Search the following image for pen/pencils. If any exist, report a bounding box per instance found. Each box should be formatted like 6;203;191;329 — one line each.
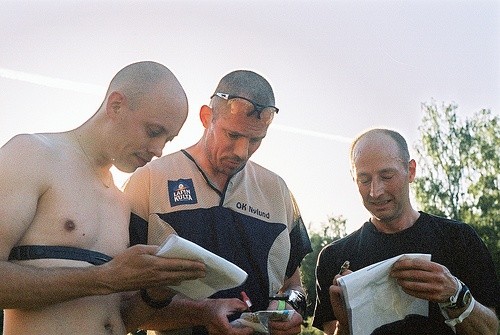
339;261;350;275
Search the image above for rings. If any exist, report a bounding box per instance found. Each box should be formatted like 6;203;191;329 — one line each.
277;299;285;310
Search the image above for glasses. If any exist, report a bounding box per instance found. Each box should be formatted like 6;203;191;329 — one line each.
211;93;280;126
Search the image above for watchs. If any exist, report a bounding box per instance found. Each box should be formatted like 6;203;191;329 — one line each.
438;276;473;309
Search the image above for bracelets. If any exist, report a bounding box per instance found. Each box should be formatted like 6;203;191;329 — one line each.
444;296;477;327
438;277;464;309
140;286;173;309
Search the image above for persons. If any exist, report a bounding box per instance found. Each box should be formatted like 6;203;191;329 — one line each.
311;128;500;335
119;70;313;335
1;61;207;335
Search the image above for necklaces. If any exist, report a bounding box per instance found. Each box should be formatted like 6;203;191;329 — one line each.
72;128;114;189
